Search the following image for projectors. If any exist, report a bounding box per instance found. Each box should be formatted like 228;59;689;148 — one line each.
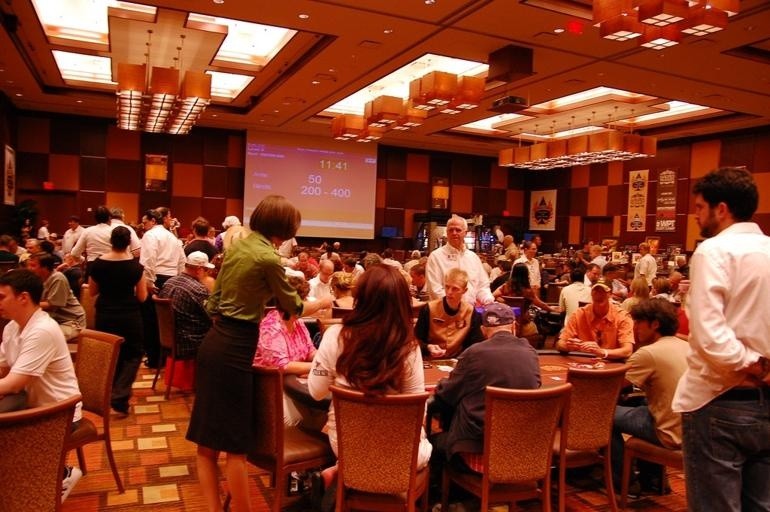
492;96;529;112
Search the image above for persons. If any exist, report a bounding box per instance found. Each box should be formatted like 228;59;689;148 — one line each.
14;218;64;270
140;211;187;369
422;218;495;306
492;263;561;337
1;269;82;503
89;226;147;415
544;260;575;288
71;207;115;284
362;247;432;292
533;235;543;255
184;217;218;262
324;271;357;319
302;259;335;349
156;207;171;229
556;282;635;394
510;241;540;298
158;251;215;361
111;208;141;258
63;216;87;253
278;236;369;279
308;264;431;509
414;267;487;361
559;270;593;326
600;298;692;498
0;235;19;269
494;224;519;280
27;251;86;340
186;195;335;512
253;276;329;493
431;303;542;511
671;168;769;511
577;240;629;296
620;245;690;312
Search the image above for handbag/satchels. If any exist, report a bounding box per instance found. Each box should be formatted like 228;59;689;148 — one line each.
528;304;542;321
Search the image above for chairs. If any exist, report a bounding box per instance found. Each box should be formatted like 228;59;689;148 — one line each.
413;302;427;318
328;384;429;512
65;283;100;363
66;328;126;494
547;283;567;302
502;296;539;348
332;307;353;318
441;383;572;512
620;432;684;509
553;364;633;512
303;318;324;350
0;394;83;512
222;363;336;512
0;261;17;272
151;294;197;399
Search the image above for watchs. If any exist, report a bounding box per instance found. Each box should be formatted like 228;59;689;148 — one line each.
602;349;608;358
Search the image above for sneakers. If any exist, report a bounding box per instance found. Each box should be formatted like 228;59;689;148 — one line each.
431;502;466;512
487;503;508;511
637;485;662;495
614;485;638;498
59;466;82;503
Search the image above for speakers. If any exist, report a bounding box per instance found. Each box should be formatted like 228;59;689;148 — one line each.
488;44;534;83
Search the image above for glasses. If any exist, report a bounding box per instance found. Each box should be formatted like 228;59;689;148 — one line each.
201;266;209;271
596;328;603;347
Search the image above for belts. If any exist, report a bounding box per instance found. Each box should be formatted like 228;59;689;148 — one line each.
713;384;769;403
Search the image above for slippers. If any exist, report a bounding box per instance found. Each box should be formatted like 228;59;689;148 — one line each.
309;470;324;510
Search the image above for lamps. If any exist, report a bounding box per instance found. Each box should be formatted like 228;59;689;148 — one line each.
364;96;403;129
491;93;671;170
105;6;229;135
592;0;739;51
438;76;485;116
408;71;457;111
392;101;428;131
354;127;385;143
329;114;364;143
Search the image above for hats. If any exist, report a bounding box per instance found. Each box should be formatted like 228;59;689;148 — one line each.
481;301;516;327
592;275;614;292
185;250;216;269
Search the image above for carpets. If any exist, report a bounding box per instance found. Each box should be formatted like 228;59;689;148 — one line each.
63;355;308;511
452;465;688;512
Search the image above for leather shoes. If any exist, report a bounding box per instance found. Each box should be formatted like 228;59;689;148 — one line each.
145;359;151;366
